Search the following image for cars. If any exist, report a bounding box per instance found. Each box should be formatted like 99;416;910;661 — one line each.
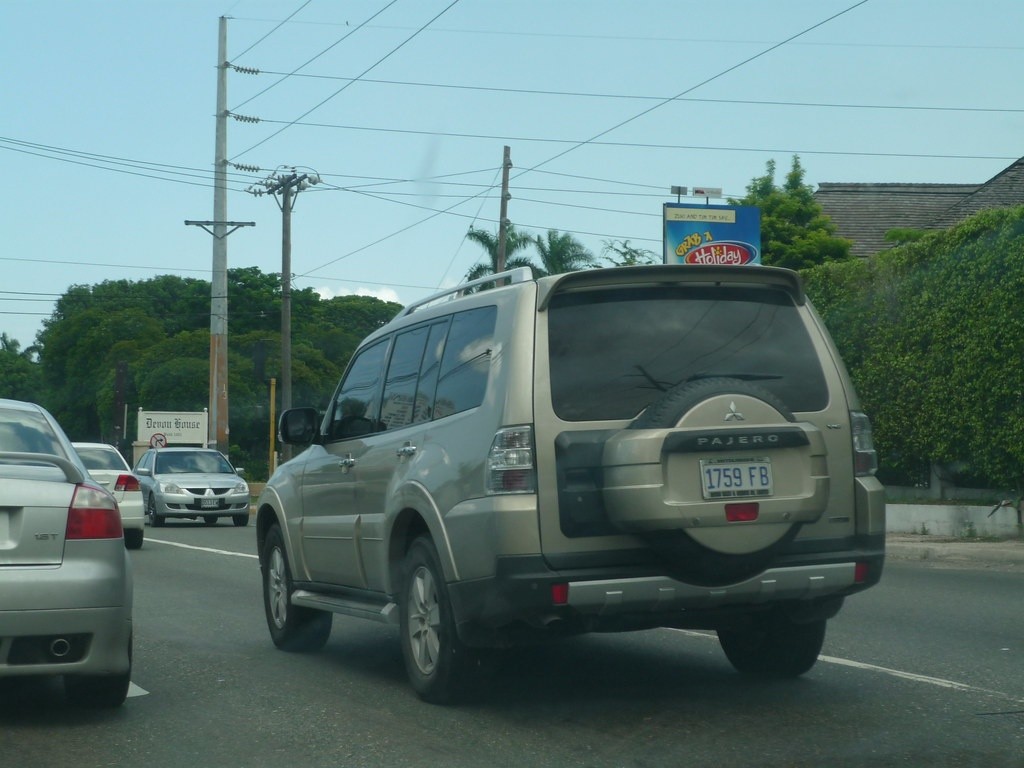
0;394;139;709
130;446;253;529
63;441;147;550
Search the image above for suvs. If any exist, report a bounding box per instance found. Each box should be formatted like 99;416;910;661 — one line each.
253;261;889;707
